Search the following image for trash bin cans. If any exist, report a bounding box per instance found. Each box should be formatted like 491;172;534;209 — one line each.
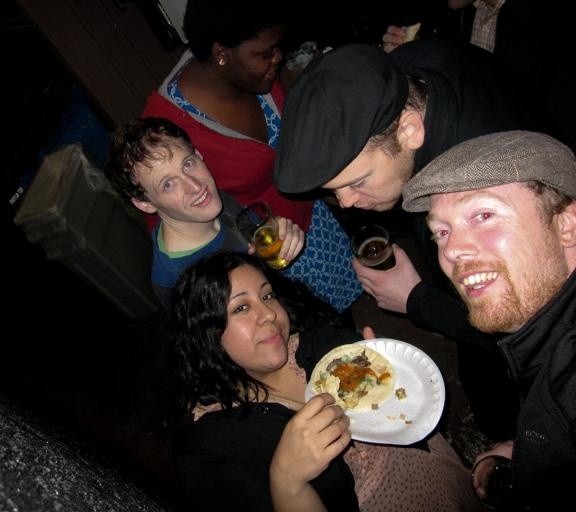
13;144;159;317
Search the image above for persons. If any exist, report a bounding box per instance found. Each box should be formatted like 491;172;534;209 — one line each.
107;1;576;512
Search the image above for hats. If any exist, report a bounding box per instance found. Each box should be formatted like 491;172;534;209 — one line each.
400;131;575;214
272;44;409;194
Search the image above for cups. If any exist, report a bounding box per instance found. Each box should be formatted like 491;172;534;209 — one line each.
350;224;395;270
234;201;291;272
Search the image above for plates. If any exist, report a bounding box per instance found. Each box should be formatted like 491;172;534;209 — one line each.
303;338;446;447
